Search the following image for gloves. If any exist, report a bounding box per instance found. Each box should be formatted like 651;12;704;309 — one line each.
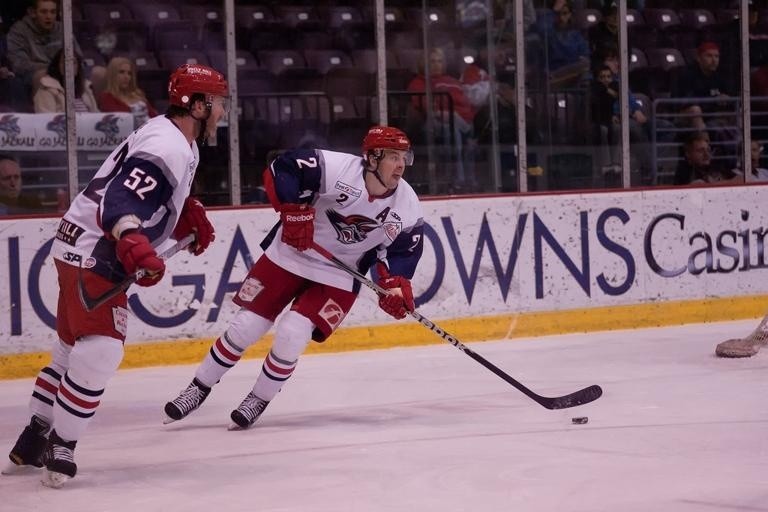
173;194;217;259
278;205;318;253
115;231;168;289
377;274;417;321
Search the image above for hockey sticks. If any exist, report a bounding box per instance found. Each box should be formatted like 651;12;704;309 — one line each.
77;233;195;310
311;242;602;410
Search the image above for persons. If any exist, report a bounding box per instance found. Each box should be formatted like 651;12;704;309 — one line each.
5;64;233;480
0;18;18;80
405;0;768;186
161;124;429;427
31;47;102;116
5;0;89;77
96;56;159;119
0;154;48;215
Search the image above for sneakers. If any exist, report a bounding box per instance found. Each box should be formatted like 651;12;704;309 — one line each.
163;376;221;421
42;427;81;477
8;414;52;469
228;389;272;430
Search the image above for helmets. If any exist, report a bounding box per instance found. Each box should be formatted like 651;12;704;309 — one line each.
165;62;230;112
358;123;413;158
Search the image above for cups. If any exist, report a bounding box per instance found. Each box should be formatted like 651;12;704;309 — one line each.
54;187;70;212
